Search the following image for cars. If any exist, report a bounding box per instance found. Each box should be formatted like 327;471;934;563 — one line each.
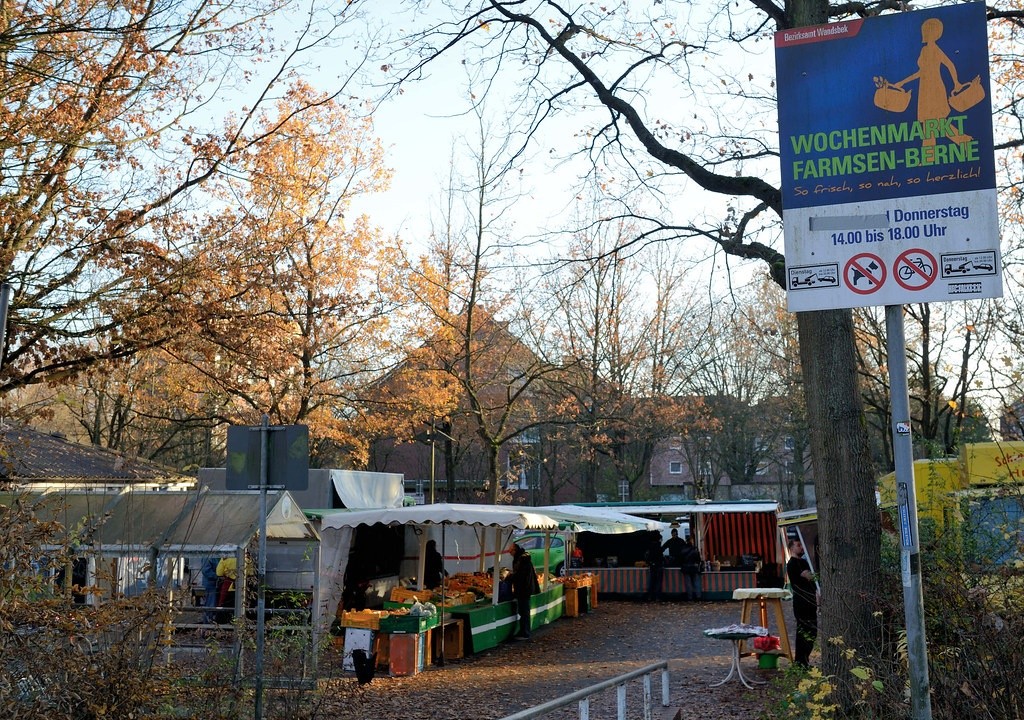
512;532;566;578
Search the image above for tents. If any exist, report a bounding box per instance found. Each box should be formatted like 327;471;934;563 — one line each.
537;503;672;575
312;501;559;663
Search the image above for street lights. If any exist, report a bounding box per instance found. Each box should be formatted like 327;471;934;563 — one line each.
413;416;455;503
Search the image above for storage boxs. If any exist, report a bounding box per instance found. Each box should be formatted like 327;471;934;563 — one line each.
340;608;388;631
565;574;599;589
379;614;429;634
427;612;441;628
389;586;433;604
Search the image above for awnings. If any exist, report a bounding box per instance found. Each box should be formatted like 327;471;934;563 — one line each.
778;505;820;591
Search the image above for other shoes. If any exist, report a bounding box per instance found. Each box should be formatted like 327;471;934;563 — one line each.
513;632;529;640
793;664;815;673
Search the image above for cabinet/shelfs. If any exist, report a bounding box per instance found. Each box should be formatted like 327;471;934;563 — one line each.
570;567;756;593
383;582;564;655
389;618;464;676
566;584;599;617
702;623;771;690
342;627;373;671
733;585;794;668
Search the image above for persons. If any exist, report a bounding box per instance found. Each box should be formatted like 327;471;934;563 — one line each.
649;535;664;603
787;540;817;667
199;557;220;638
424;540;448;589
341;575;372;611
215;551;256;629
678;535;703;603
507;543;542;641
662;528;687;565
